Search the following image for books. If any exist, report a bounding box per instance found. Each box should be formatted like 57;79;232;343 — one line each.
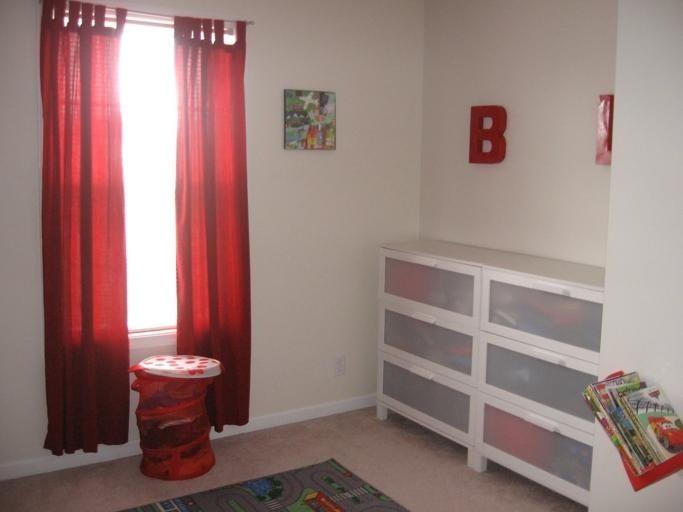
581;370;683;477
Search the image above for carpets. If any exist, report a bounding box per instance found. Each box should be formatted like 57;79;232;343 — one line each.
117;458;411;512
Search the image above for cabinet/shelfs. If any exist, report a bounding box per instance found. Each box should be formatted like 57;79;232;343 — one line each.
375;241;604;506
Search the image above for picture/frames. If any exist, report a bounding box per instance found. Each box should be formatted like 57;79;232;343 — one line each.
283;90;336;151
